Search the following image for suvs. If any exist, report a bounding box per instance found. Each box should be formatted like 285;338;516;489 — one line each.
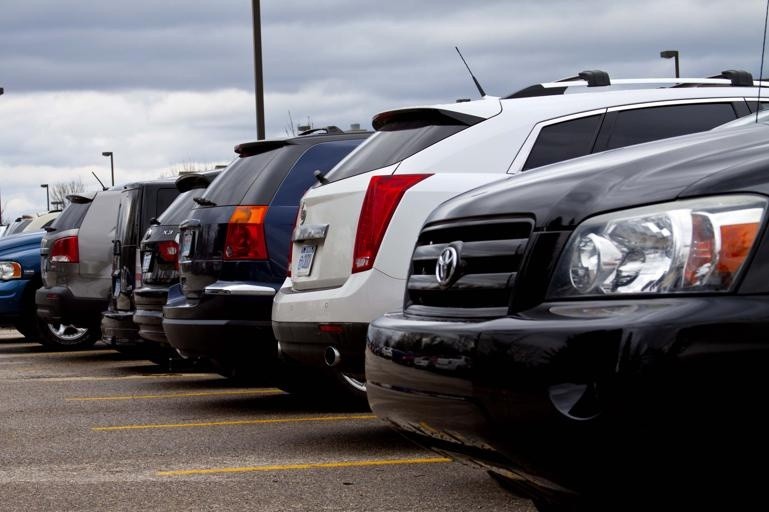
364;108;767;507
268;68;769;507
162;129;371;398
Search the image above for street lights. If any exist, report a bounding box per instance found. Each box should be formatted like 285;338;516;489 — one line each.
101;151;115;187
40;182;50;211
